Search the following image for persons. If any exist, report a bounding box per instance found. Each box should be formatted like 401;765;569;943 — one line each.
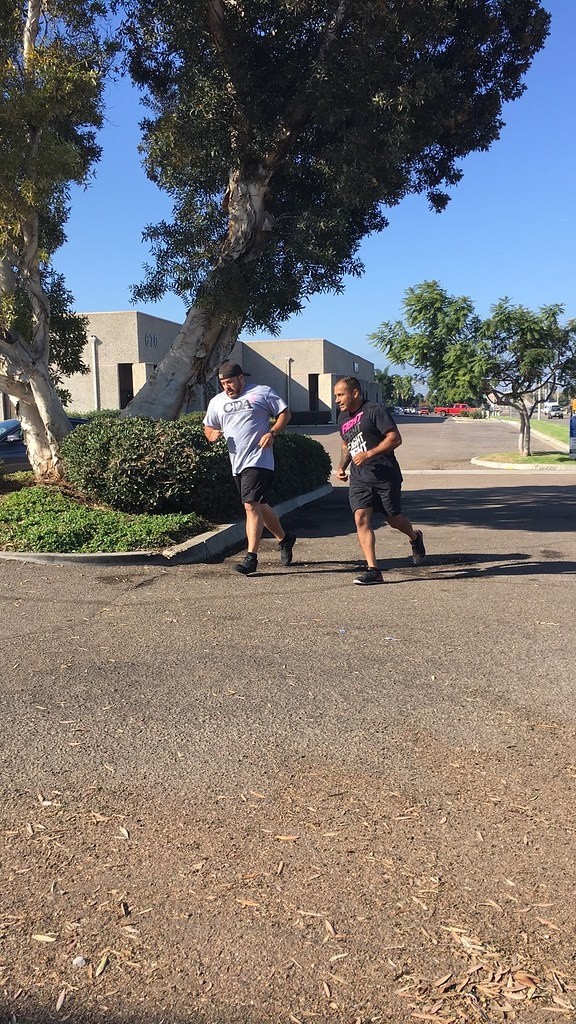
201;361;297;574
332;375;426;586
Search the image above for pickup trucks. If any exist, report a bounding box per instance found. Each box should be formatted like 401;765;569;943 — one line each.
435;403;479;417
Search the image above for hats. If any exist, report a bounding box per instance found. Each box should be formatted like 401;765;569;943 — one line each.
219;362;252;380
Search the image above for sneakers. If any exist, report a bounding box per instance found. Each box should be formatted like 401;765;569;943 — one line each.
410;530;425;565
232;555;258;574
278;534;296;566
353;568;384;584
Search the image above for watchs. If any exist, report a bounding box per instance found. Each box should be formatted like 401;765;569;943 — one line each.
268;429;279;440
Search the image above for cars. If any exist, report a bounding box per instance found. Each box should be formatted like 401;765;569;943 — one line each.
420;406;429;415
404;407;416;414
394;407;404;414
0;418;93;474
529;405;538;413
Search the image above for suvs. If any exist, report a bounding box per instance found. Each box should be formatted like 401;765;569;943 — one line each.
548;405;563;419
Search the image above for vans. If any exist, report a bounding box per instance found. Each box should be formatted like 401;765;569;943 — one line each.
542;403;560;415
